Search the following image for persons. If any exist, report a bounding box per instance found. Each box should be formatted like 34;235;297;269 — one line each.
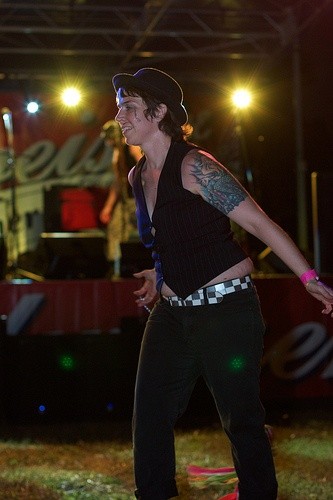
113;67;333;500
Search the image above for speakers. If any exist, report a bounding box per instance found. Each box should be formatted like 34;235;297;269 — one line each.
119;242;155;279
47;234;108;280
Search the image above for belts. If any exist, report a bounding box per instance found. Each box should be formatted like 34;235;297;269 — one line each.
162;275;253;306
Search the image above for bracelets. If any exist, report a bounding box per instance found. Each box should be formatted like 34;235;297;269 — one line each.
300;269;319;286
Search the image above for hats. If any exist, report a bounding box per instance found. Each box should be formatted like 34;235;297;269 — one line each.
112;68;189;127
